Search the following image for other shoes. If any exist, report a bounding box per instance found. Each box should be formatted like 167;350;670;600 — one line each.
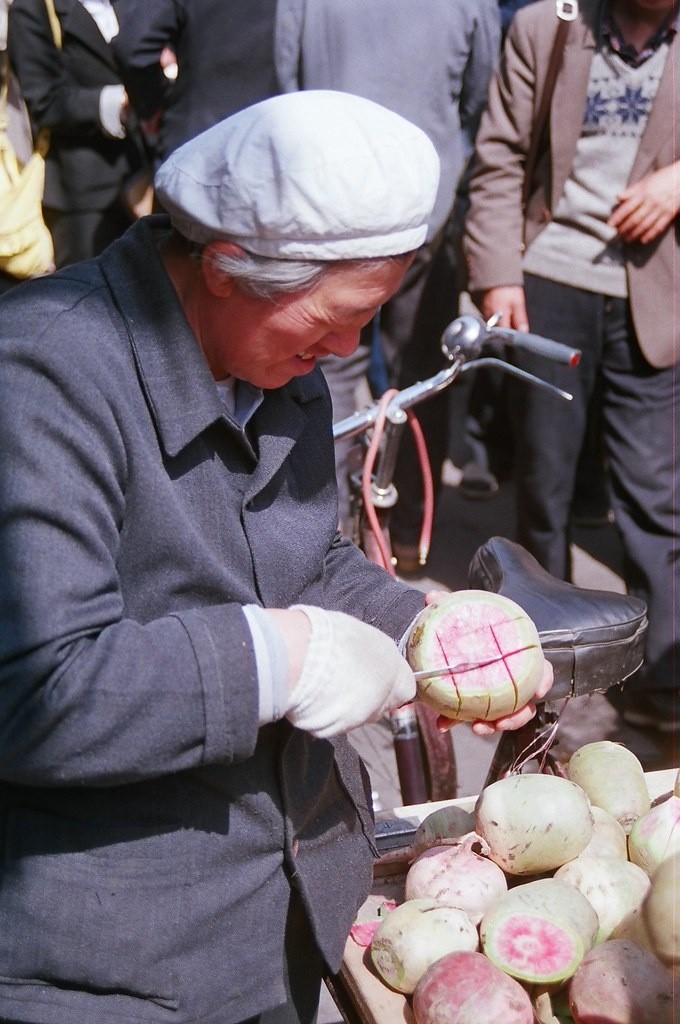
391;540;424;573
622;690;680;732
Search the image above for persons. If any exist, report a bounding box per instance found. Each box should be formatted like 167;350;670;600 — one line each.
0;91;554;1024
0;0;680;735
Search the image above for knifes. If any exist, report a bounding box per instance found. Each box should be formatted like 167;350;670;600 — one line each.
416;656;510;677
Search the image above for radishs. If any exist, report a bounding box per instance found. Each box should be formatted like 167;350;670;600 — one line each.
368;740;680;1024
407;590;544;722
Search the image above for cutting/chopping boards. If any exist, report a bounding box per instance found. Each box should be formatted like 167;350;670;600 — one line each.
342;767;680;1024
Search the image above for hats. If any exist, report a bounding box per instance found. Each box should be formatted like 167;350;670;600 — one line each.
154;90;441;260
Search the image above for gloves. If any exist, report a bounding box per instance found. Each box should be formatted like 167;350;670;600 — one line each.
282;604;417;739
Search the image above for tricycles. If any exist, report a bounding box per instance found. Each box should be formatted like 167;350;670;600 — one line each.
323;309;679;1024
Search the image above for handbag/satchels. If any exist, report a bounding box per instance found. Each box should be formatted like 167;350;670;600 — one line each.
1;115;56;279
